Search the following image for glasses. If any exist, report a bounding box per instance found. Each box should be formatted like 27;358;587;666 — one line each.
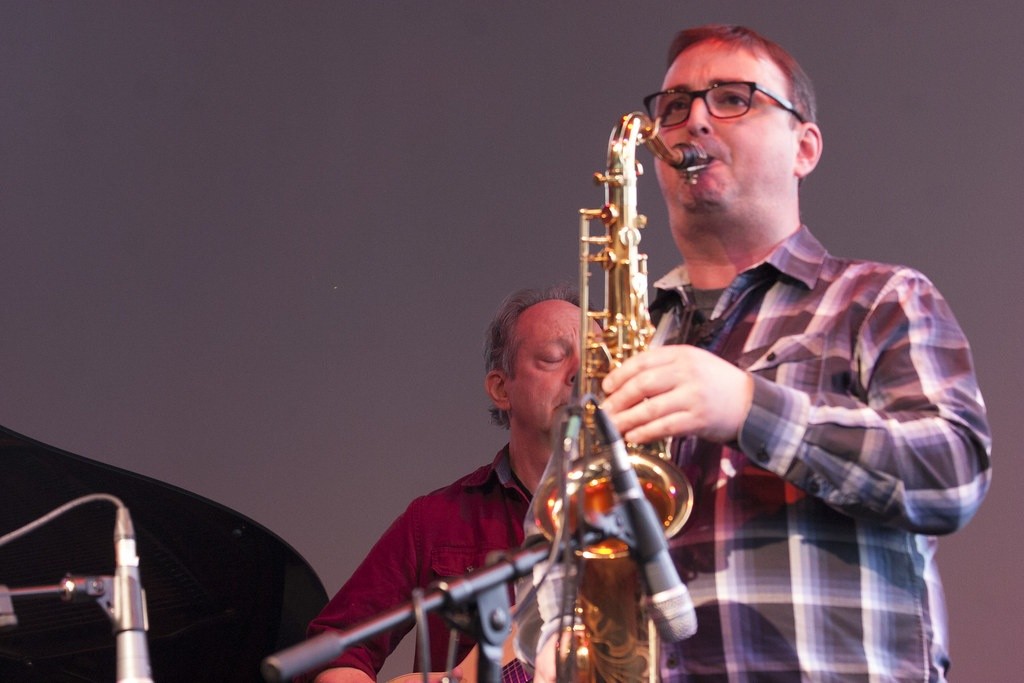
642;82;807;129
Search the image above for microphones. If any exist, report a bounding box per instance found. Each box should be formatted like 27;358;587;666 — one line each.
594;409;697;644
113;507;155;683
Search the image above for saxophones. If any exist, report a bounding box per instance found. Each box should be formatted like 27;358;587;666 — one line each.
551;110;710;681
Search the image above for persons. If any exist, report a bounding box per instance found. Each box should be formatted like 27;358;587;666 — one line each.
295;284;602;682
601;24;993;683
